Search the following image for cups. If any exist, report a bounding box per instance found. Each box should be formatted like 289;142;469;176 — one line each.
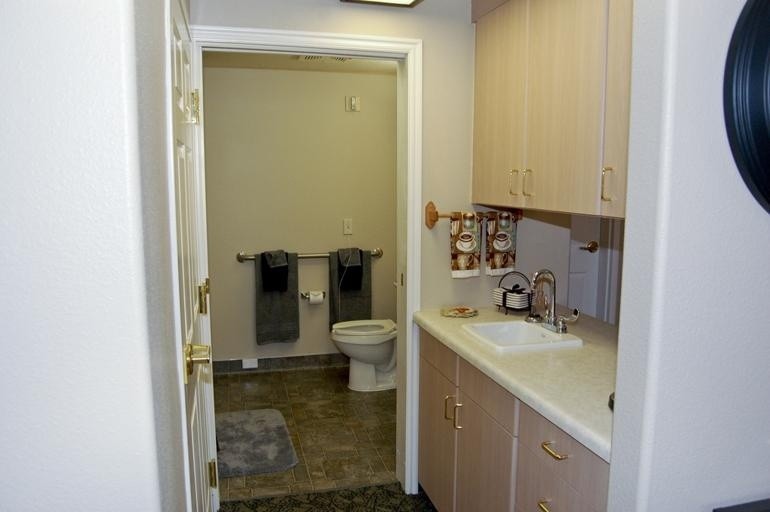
458;253;473;269
494;253;507;269
459;232;475;247
495;231;511;247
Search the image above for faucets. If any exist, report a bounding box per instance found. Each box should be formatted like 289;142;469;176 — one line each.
530;268;557;332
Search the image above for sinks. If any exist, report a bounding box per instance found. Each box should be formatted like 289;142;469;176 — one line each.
462;320;584;352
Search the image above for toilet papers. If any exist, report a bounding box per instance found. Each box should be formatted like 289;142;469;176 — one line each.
309;291;323;305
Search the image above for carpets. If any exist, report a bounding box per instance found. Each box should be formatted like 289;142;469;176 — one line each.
215;408;300;479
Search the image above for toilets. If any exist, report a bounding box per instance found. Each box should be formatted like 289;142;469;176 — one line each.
331;280;398;392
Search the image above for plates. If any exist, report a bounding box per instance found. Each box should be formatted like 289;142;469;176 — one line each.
491;287;536;309
493;240;511;251
456;240;477;252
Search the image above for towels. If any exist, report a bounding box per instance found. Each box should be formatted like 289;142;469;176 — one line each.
449;211;517;279
328;247;372;333
253;248;300;346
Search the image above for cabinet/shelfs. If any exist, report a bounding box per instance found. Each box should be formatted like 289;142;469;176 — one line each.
418;326;611;512
470;0;607;217
600;1;634;219
